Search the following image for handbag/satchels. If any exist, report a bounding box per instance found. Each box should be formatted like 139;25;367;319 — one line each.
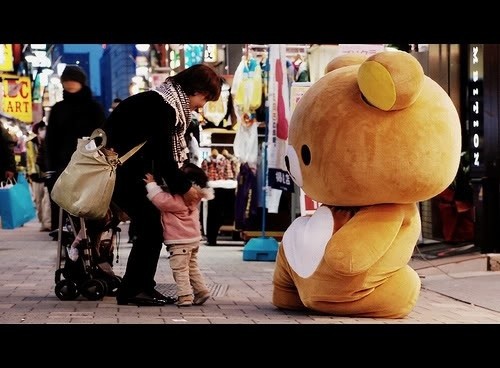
51;128;119;220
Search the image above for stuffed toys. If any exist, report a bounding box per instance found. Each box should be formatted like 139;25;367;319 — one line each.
271;49;462;320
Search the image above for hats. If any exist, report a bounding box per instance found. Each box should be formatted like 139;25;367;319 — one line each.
33;120;43;134
61;66;87;85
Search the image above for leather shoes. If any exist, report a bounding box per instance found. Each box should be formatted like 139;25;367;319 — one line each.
148;288;177;304
116;290;168;305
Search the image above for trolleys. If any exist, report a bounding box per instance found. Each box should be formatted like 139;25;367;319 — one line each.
54;199;123;302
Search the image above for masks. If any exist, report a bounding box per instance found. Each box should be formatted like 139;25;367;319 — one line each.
38;130;47;137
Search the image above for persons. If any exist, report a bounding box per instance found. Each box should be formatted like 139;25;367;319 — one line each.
0;120;17;186
34;64;105;261
26;121;52;232
94;64;221;305
142;163;215;307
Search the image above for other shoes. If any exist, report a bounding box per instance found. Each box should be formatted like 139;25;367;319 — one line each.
195;289;210;306
177;293;194;307
